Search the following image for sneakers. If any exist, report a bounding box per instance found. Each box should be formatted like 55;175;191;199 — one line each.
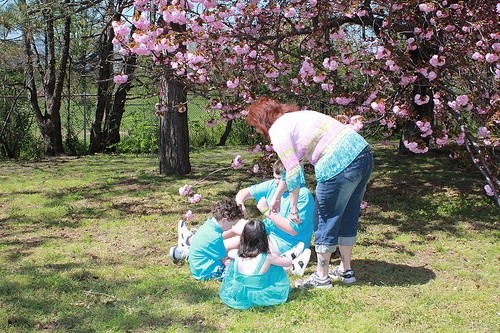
178;220;193;247
290;249;311;275
328;266;356;283
170;247;190;261
295;273;332;289
280;242;305;269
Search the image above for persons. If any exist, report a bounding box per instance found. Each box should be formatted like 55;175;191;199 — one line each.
169;160;316;263
219;219;292;310
189;198;311;281
246;97;375;291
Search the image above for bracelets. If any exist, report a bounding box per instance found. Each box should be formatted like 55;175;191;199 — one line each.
261;208;269;215
